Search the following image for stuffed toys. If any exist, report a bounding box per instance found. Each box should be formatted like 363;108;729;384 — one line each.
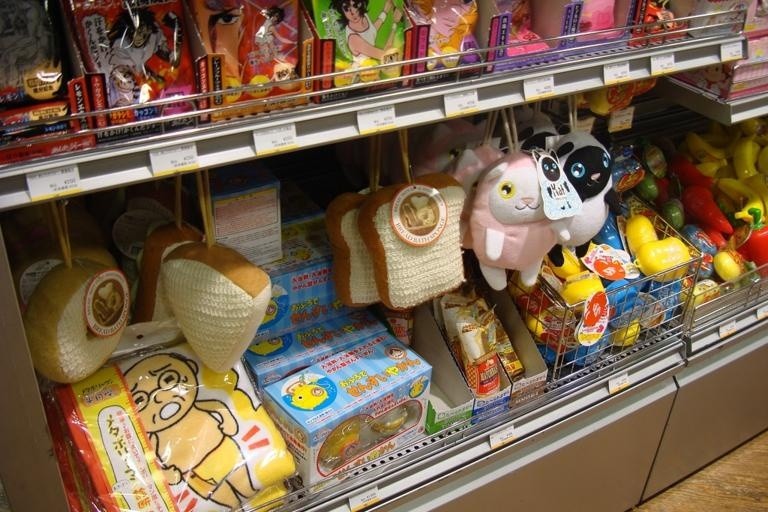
414;97;622;291
417;107;573;264
548;130;622;266
471;153;570;291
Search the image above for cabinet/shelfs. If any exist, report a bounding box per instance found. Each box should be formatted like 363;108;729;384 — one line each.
1;13;767;511
